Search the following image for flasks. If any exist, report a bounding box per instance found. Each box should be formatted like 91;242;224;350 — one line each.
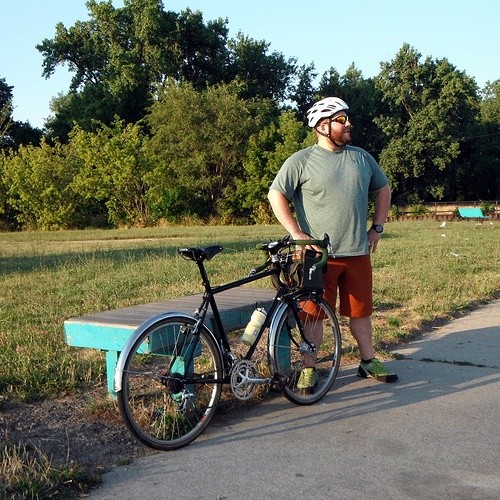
241;300;267;346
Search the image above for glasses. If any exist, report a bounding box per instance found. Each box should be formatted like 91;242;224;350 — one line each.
324;115;348;124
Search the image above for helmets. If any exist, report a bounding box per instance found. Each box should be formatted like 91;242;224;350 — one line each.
307;97;349;127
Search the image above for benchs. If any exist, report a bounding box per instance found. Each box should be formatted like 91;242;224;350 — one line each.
64;287;296;401
458;207;491;223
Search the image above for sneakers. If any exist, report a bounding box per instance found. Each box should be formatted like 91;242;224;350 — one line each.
357;357;398;383
297;367;318;394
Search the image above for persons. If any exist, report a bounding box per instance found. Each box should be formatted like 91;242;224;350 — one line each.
267;97;399;395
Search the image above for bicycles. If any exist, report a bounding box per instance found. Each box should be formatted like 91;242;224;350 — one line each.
113;233;341;451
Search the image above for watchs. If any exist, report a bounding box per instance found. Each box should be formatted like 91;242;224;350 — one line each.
371;224;383;233
